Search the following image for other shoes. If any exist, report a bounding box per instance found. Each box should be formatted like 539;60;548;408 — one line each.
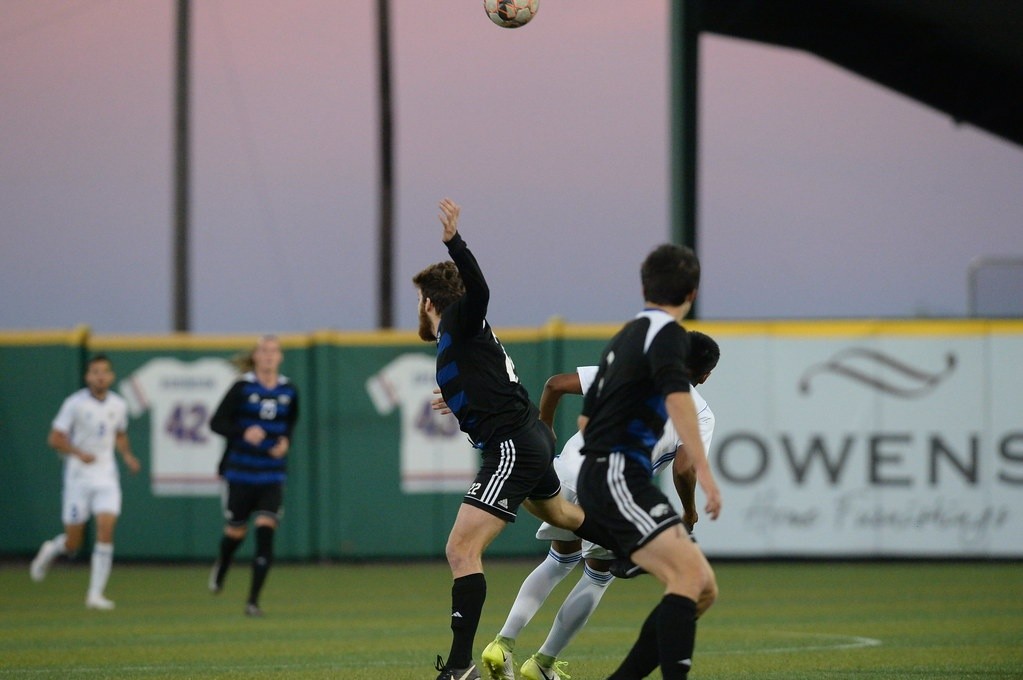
243;601;263;620
84;593;117;611
30;540;50;580
207;563;225;594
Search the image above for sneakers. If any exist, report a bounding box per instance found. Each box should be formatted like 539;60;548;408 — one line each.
434;655;481;680
519;653;572;680
481;642;516;680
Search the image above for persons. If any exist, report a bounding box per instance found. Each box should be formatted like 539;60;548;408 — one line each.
211;336;298;617
412;196;722;680
31;327;139;611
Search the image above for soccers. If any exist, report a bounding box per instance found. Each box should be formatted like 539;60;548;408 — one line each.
483;0;540;29
